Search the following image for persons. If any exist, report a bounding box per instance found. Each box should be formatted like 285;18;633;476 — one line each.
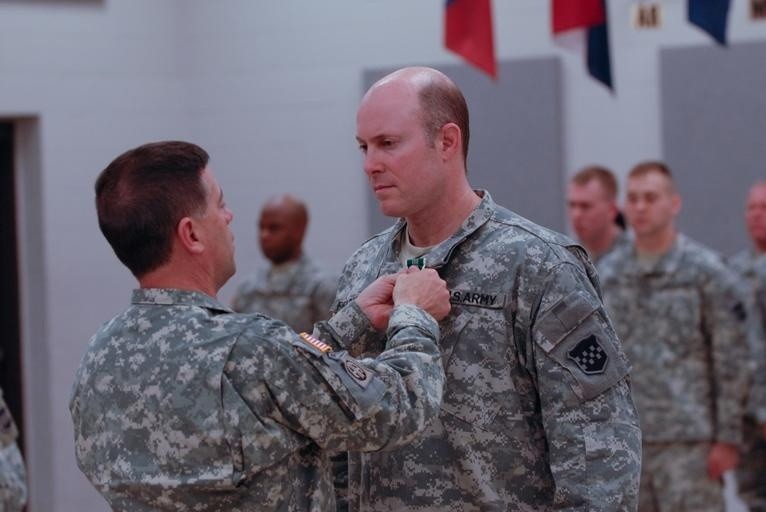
328;64;645;510
224;193;339;342
586;157;760;512
704;173;766;512
562;162;629;265
64;136;453;512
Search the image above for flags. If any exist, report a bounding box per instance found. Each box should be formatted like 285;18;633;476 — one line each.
685;1;732;50
548;1;616;90
440;1;500;77
